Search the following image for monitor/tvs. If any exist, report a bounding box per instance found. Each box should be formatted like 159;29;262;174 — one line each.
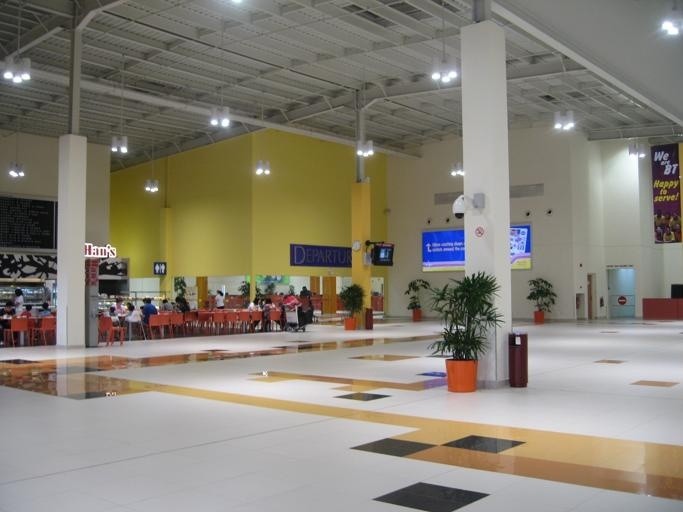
372;243;395;266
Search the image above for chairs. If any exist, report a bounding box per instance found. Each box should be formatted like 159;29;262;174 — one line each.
3;315;56;347
98;306;300;346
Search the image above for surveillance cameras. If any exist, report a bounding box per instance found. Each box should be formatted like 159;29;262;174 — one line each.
452;197;466;219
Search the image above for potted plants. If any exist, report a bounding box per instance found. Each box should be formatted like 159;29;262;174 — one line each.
403;279;430;320
425;272;505;393
337;284;365;330
525;277;558;323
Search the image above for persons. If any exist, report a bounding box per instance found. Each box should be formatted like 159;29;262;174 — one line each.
38;302;51;316
107;296;191;339
19;304;31;318
299;286;311;295
0;301;17;345
214;290;224;308
248;293;312;334
12;288;23;312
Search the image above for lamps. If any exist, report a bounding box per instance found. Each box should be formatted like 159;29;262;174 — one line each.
144;140;159;193
8;124;25;178
3;6;31;84
256;103;270;176
110;56;128;155
450;130;464;176
659;0;680;35
210;25;230;128
629;111;647;159
357;52;374;158
431;0;458;83
553;65;575;130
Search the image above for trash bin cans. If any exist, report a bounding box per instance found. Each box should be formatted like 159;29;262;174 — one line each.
509;333;528;387
366;308;373;330
